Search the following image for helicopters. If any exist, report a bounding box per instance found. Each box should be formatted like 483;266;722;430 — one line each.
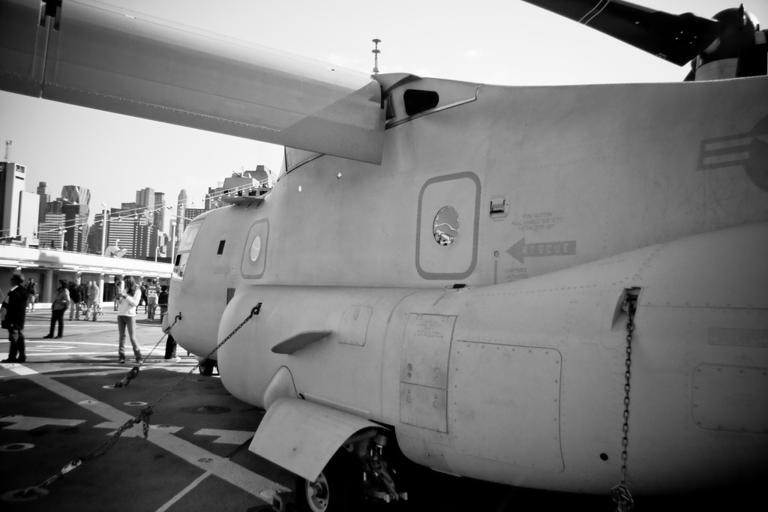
1;1;768;511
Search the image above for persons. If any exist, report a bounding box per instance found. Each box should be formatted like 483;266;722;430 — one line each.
24;277;38;313
114;275;144;364
43;279;71;341
163;334;182;363
64;273;174;322
0;273;31;363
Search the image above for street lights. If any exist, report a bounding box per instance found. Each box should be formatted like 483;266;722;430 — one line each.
114;237;120;248
57;227;70;253
171;220;177;265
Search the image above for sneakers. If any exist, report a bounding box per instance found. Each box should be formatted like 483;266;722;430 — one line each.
43;334;63;340
0;354;27;364
164;356;182;363
137;360;144;364
68;317;97;322
118;356;125;363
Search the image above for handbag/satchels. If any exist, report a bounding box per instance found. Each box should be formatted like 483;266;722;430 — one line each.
50;299;68;311
